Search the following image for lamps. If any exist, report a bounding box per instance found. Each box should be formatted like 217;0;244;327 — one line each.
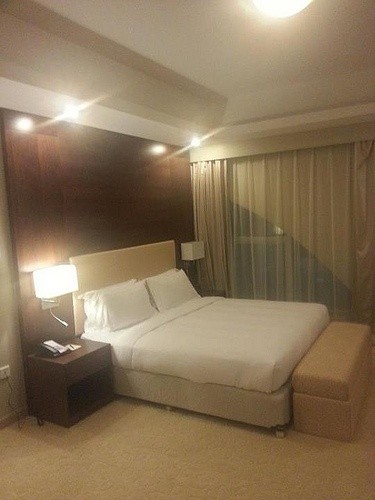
181;241;205;274
33;264;79;303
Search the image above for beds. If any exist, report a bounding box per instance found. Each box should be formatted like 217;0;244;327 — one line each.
69;240;330;429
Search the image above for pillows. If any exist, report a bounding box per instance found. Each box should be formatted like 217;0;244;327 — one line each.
77;279;136;323
138;268;202;313
93;280;158;331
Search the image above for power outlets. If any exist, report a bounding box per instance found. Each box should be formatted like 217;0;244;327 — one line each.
41;297;58;311
0;365;11;380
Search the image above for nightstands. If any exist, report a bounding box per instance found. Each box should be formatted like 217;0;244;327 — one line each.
26;338;114;428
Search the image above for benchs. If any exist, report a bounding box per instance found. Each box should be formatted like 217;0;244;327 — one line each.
291;321;373;443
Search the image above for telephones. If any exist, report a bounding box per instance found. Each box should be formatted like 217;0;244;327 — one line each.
38;339;71;359
